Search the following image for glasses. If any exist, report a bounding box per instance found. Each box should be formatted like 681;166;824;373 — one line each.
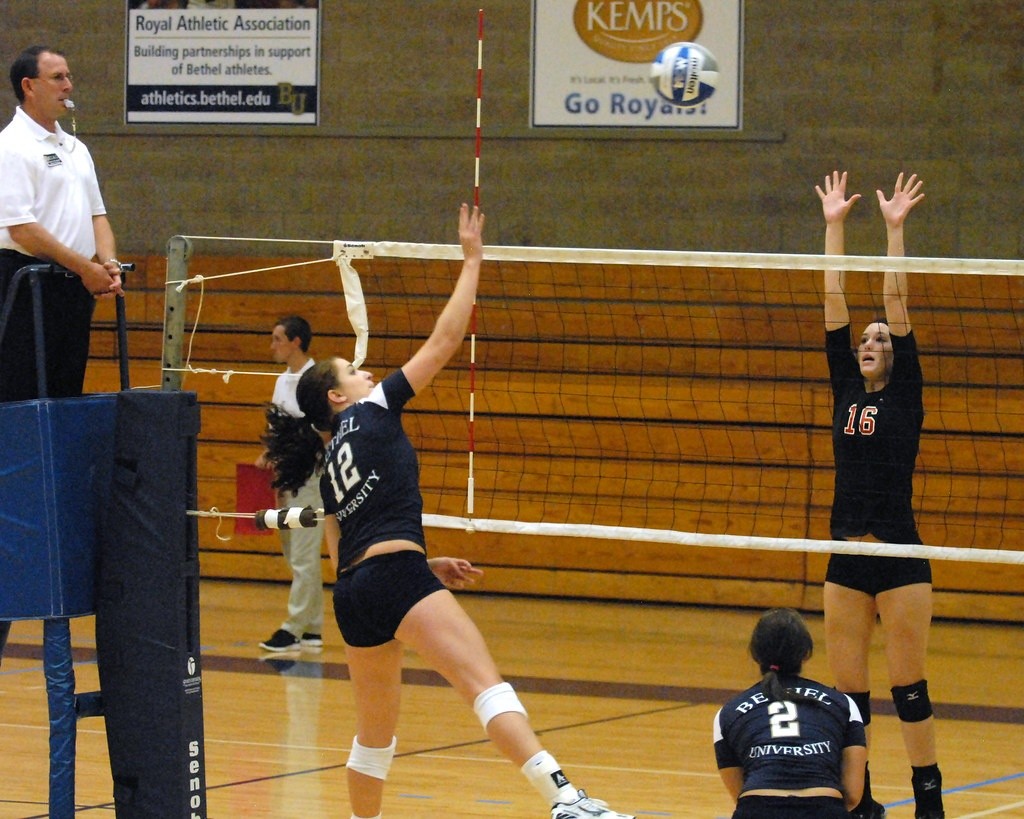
34;71;75;85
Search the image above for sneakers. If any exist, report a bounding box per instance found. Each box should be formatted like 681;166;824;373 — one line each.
259;628;322;652
552;793;632;819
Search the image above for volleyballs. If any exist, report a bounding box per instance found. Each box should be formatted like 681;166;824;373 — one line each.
649;40;720;109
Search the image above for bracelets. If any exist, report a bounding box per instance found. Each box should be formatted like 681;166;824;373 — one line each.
110;259;122;270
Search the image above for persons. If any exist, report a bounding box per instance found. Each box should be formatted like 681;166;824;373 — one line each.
254;316;326;652
815;170;945;819
713;606;867;819
0;46;125;404
260;203;635;819
